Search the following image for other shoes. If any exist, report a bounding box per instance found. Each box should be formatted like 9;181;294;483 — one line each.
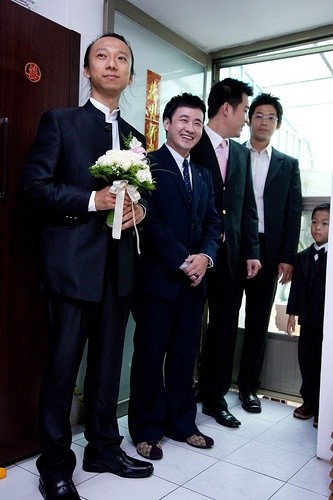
137;439;164;459
293;403;313;419
312;414;318;427
186;430;214;447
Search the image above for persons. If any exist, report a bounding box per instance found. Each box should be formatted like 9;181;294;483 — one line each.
24;33;302;500
286;203;331;428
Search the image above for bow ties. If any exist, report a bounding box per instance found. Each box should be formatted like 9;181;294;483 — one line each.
314;247;325;259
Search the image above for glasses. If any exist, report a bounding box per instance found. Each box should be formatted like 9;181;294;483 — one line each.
251;114;278;121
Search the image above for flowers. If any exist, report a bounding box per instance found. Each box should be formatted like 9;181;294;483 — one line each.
86;131;155;226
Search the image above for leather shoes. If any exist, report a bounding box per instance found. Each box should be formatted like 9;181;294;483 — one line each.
82;447;154;477
38;476;81;500
202;406;241;427
239;391;261;412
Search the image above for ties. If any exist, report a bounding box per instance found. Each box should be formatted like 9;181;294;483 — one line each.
181;159;192;204
216;140;227;182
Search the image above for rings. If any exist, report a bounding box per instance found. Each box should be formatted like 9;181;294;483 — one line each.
193;273;199;279
